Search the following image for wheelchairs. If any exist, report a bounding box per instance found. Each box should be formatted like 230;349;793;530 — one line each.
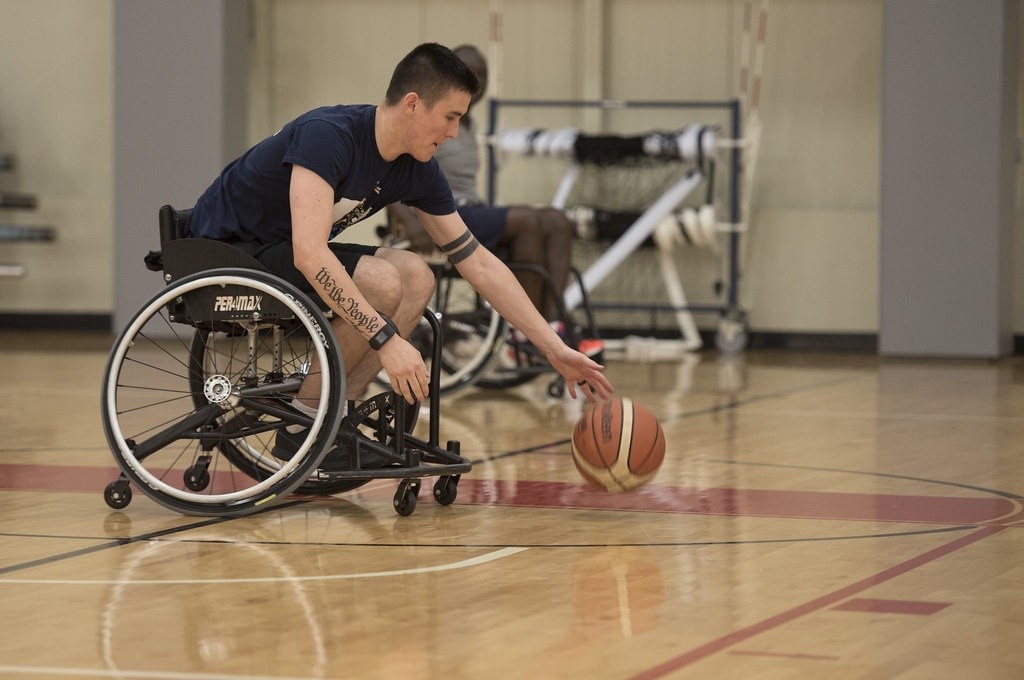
102;204;472;519
373;200;604;398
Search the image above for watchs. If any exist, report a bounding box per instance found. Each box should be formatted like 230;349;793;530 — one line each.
368;324;395;351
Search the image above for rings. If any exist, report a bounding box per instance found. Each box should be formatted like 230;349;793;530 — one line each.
578;379;586;387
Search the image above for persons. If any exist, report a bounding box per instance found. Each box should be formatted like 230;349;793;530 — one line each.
181;43;613;473
385;44;606;366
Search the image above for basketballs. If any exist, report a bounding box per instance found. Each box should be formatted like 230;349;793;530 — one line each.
570;396;665;494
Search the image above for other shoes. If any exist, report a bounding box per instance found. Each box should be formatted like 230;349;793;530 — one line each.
506;320;604;361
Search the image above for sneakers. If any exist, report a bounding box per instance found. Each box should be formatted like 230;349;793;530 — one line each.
271;421;396;470
